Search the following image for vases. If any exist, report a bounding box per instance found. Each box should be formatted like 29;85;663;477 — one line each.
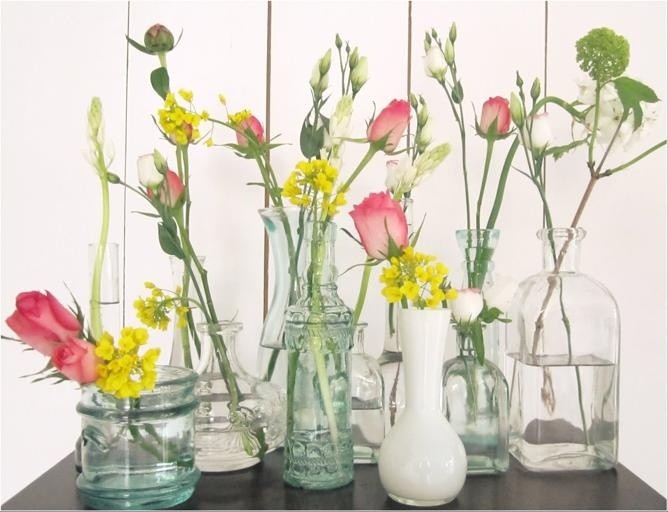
506;227;622;472
377;307;467;506
76;364;201;508
442;229;510;477
168;256;206;366
89;242;124;349
258;207;404;490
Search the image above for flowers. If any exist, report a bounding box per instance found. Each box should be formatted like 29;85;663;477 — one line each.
106;23;662;463
82;96;116;334
1;281;177;458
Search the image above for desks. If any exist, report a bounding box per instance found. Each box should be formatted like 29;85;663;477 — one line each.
0;430;666;512
